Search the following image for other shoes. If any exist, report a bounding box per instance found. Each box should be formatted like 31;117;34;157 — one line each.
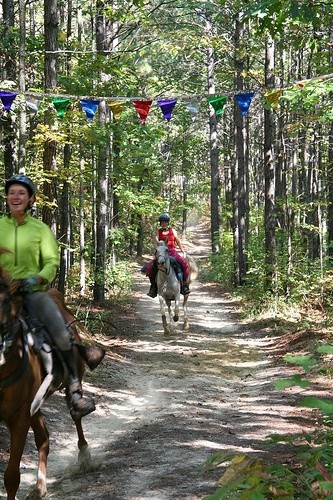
147;286;157;298
181;282;190;292
65;392;94;416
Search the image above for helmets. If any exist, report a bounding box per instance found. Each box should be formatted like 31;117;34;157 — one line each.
5;174;36;197
159;214;170;222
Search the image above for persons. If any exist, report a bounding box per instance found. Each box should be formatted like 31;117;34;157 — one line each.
0;174;95;420
147;214;191;296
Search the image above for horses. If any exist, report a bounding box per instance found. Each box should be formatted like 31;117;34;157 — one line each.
0;246;93;500
155;237;192;334
